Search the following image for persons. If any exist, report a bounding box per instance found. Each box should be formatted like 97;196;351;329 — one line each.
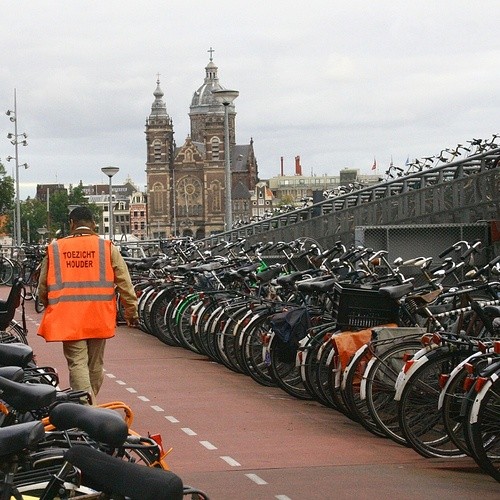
36;207;140;406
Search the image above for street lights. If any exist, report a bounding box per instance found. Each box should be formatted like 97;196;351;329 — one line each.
214;84;239;232
5;81;30;250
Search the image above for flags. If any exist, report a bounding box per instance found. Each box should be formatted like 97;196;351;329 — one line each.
390;158;393;168
405;156;409;166
372;159;376;170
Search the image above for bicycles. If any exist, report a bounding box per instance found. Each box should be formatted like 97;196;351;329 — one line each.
0;133;500;500
